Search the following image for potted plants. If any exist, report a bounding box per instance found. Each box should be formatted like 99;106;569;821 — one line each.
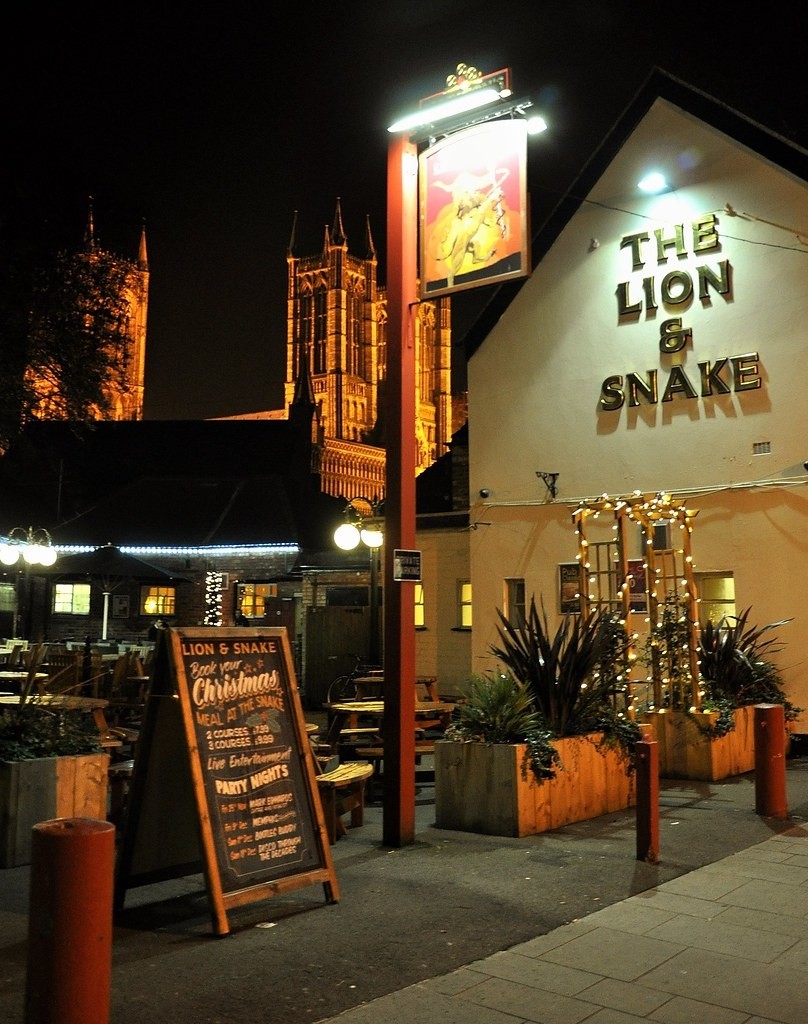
436;587;807;838
0;623;111;869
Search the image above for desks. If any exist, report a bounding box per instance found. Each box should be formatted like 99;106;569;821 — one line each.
0;671;49;696
39;662;108;686
90;642;137;652
353;677;439;702
0;693;110;738
323;702;459;743
0;649;32;671
305;724;323;775
100;654;145;668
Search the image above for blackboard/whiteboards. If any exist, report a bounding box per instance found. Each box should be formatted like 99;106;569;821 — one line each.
169;625;333;902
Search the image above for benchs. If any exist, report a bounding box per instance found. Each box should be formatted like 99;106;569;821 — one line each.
97;726;140;830
316;696;441;843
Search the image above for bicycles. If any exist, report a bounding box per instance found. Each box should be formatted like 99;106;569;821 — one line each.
327;655;367;703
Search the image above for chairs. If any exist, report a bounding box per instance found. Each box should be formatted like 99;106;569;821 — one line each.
6;640;150;696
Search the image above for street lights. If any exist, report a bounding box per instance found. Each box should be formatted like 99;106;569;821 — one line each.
337;520;383;666
0;525;58;644
383;84;546;850
